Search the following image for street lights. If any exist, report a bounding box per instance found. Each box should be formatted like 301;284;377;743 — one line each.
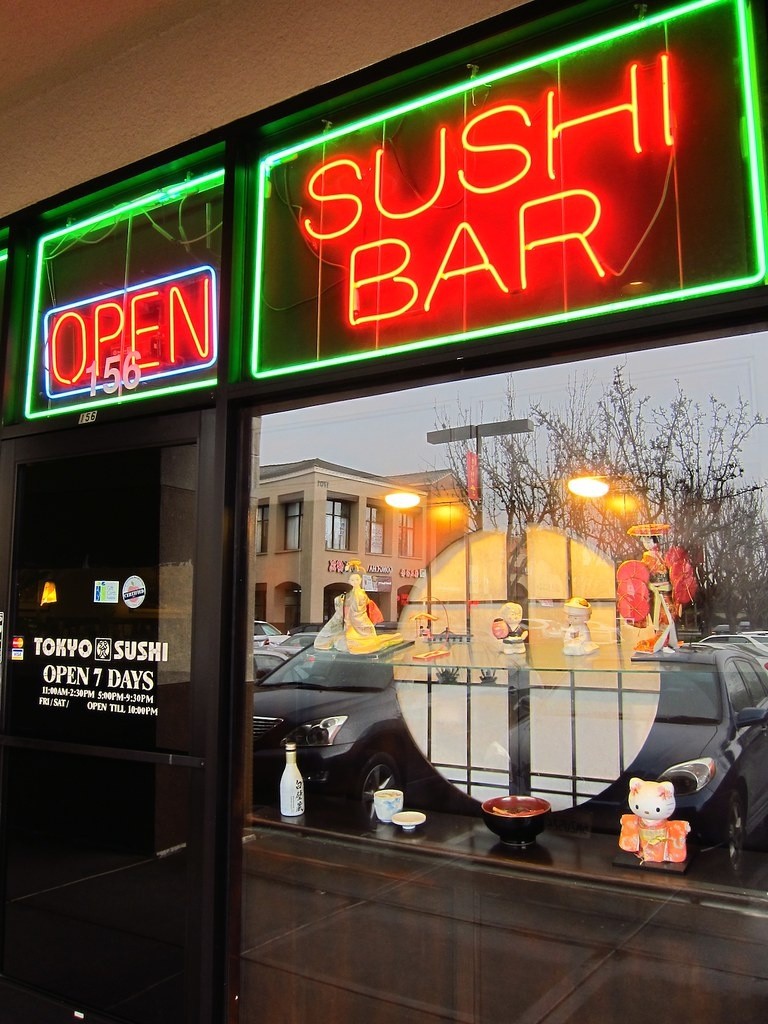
425;419;536;533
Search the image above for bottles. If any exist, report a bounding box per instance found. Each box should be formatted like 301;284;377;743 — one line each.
279;742;305;816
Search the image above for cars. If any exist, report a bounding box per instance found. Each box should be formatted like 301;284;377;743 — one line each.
563;646;768;868
252;621;327;681
253;632;464;814
680;631;767;668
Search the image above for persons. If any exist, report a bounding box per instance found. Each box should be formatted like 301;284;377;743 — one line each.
313;556;404;654
634;534;682;654
498;602;528;655
561;597;599;655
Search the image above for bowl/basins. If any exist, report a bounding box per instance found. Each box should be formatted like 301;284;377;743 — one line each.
481;795;551;846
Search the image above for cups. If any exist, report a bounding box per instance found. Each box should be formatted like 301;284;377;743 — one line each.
374;789;404;823
